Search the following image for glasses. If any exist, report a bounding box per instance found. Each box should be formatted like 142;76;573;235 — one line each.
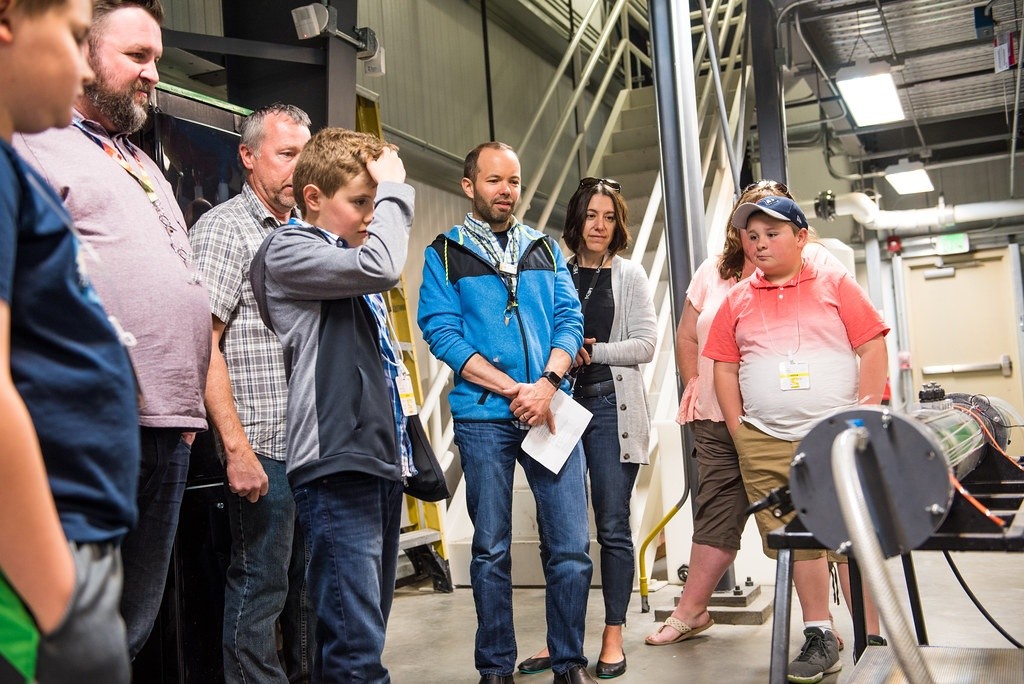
738;179;793;205
577;176;621;196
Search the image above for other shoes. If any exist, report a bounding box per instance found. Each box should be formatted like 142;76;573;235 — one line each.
518;649;553;673
595;643;627;678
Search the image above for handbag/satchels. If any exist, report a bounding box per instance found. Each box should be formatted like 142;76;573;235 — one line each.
394;407;451;504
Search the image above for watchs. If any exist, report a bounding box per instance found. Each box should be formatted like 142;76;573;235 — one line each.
540;371;562;391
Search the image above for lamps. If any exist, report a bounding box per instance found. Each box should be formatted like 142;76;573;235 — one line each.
837;60;935;194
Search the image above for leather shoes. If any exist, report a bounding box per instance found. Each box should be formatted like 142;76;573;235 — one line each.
478;673;514;684
553;665;598;684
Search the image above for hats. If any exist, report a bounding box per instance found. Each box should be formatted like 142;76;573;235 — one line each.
731;197;808;233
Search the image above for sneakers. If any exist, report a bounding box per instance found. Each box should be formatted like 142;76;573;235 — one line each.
788;626;843;684
852;634;887;664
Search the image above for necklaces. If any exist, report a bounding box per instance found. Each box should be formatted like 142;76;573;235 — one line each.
466;225;517;326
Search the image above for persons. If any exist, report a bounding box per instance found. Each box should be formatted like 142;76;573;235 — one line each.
417;142;594;684
646;178;852;652
0;0;142;684
251;127;417;684
515;177;658;679
11;0;213;663
185;104;312;684
702;198;890;678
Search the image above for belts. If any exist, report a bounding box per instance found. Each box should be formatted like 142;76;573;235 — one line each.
572;380;615;400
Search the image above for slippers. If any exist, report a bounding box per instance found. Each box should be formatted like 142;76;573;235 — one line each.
645;613;714;646
830;625;845;651
